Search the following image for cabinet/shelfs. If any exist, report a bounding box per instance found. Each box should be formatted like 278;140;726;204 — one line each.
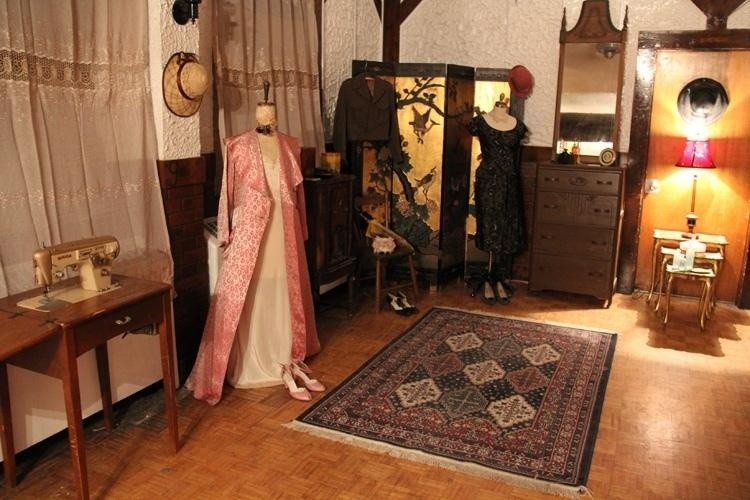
529;167;622;299
303;175;358;326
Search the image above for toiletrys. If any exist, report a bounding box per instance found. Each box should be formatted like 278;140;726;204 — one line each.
572;136;580;164
557;150;573;165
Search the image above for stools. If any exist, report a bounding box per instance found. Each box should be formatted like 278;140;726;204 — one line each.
663;263;715;333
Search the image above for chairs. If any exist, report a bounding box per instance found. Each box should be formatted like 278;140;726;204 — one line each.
354;194;419;311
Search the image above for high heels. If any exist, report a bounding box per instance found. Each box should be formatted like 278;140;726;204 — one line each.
288;358;326;392
483;281;496;306
386;291;412;317
280;364;313;402
396;290;416;315
494;281;509;305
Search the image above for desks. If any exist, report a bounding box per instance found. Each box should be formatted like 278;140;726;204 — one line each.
655;245;722;322
647;228;729;311
0;272;179;500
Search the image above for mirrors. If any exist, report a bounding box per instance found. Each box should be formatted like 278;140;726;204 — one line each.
549;0;629;166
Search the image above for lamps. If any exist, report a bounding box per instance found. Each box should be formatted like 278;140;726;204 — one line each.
674;138;716;239
596;42;621;59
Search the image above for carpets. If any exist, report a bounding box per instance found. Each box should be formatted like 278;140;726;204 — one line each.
280;305;617;499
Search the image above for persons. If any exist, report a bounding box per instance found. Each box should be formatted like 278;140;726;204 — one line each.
185;80;320;406
469;94;532;256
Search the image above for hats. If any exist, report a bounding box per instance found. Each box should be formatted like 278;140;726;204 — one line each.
508;65;536;100
161;50;210;118
677;77;728;127
